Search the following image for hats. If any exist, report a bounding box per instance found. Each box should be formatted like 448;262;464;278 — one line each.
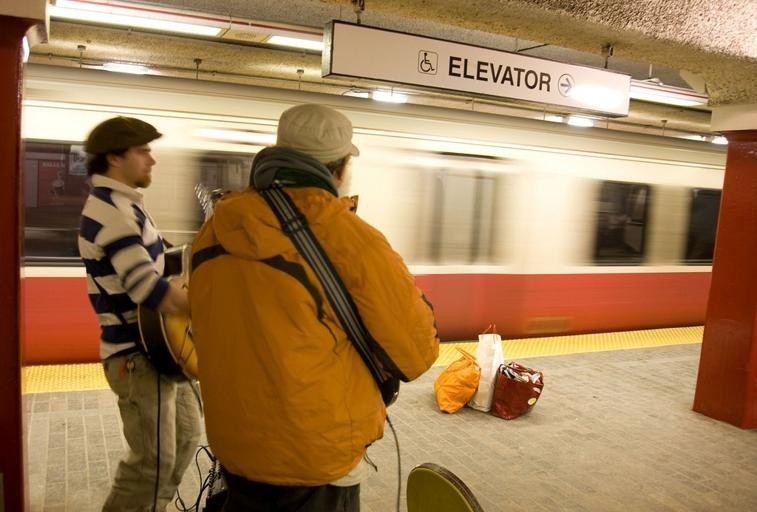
83;116;162;155
277;103;359;165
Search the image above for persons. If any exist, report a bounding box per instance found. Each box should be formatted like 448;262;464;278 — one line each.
188;102;439;512
78;114;203;512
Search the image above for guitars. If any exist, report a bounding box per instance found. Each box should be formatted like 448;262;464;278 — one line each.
138;185;229;384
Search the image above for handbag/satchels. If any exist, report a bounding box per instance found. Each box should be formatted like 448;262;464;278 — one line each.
433;322;544;420
